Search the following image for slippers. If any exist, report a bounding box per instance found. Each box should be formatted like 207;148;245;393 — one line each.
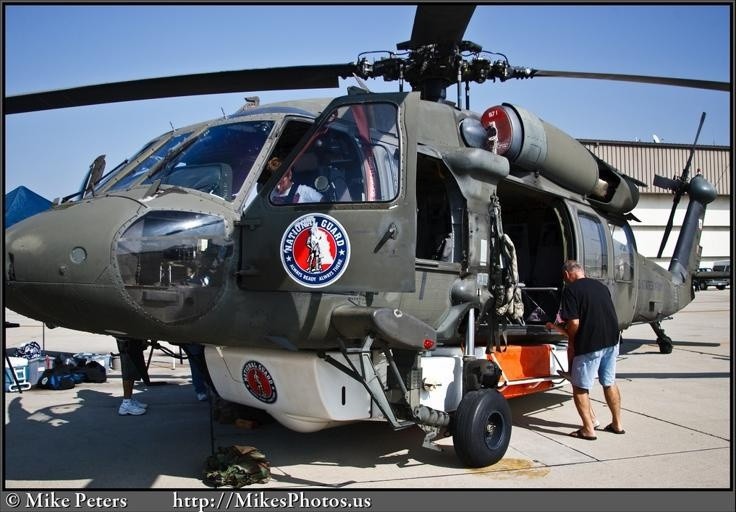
604;423;625;434
569;430;597;440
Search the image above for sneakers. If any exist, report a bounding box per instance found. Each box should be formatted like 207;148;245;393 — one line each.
118;396;148;415
197;393;207;400
557;370;573;381
593;421;600;428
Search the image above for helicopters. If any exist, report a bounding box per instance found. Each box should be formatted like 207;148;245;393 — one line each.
5;4;730;467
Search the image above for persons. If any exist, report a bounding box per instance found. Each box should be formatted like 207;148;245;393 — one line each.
547;307;600;428
117;338;148;416
560;259;623;437
187;343;208;401
262;156;327;204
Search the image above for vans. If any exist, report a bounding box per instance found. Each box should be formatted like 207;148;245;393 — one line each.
713;261;731;289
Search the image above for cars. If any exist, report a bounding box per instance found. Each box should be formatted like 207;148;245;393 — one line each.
693;268;729;290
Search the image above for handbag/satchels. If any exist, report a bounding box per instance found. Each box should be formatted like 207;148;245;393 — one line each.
203;444;270;487
38;360;105;389
